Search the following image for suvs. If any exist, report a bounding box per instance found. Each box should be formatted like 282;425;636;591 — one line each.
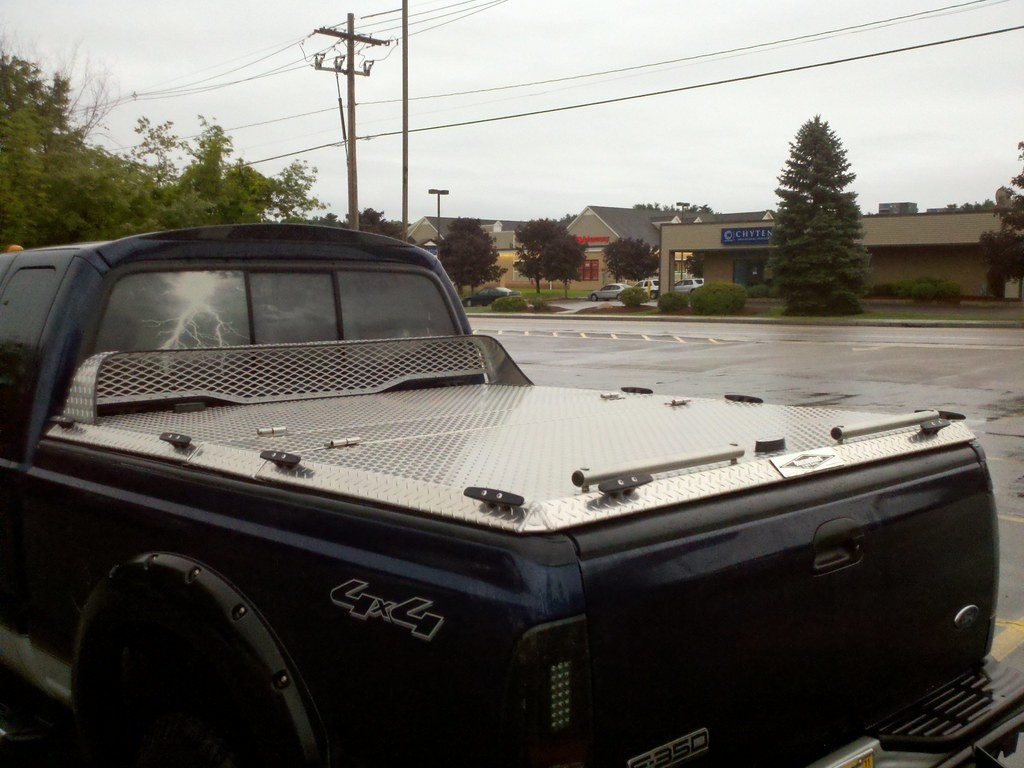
633;280;660;300
674;278;704;295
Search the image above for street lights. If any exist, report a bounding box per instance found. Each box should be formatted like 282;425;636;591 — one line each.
428;188;450;260
676;202;690;279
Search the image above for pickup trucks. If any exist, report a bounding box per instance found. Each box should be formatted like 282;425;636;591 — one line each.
1;215;1022;768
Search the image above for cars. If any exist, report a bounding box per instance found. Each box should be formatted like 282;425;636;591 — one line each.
461;286;522;307
587;283;634;301
